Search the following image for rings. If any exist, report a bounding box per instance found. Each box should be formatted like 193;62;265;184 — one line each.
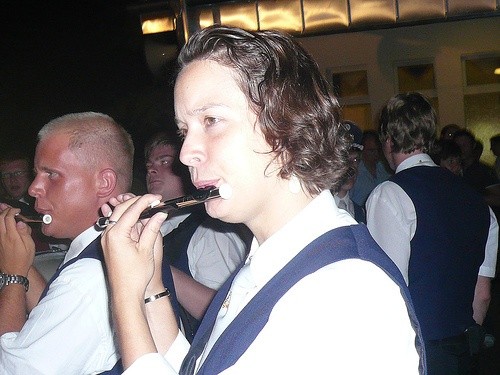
105;219;116;225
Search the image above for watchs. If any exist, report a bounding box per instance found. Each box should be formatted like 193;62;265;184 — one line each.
0;273;29;292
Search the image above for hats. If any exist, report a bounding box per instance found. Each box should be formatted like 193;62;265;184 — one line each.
339;120;365;150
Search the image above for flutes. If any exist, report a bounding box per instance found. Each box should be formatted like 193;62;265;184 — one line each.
93;183;234;232
13;213;54;226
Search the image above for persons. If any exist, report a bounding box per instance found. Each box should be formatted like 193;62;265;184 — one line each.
145;132;248;344
0;112;135;375
100;22;427;375
334;121;500;375
365;92;499;375
0;152;50;250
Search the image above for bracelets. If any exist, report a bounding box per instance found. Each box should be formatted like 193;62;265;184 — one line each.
145;288;170;304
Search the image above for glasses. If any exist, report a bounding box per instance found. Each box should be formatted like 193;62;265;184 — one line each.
351;156;361;165
2;170;36;180
147;156;177;170
